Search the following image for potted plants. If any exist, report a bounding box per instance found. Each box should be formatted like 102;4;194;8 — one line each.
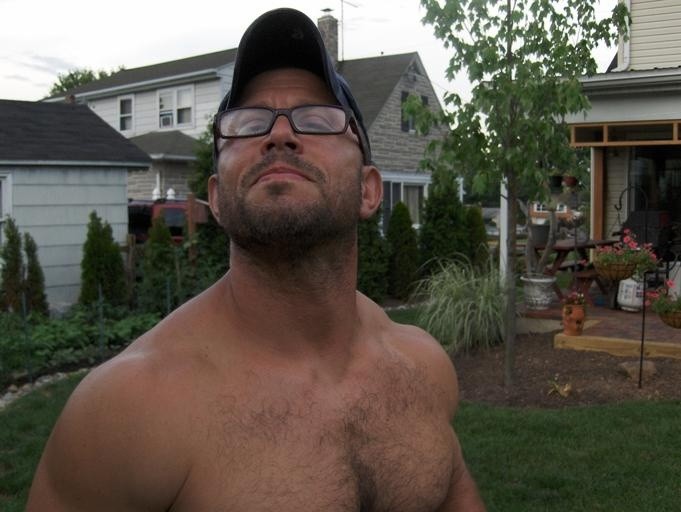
398;0;634;311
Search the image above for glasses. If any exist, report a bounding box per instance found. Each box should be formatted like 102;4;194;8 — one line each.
213;105;367;162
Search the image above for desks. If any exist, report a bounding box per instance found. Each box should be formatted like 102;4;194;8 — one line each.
514;239;621;306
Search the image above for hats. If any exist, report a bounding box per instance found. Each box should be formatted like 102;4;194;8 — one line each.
213;7;369;143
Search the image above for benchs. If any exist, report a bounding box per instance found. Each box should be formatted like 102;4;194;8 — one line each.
522;260;609;313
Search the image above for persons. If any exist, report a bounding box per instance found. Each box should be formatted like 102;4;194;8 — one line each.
24;8;489;512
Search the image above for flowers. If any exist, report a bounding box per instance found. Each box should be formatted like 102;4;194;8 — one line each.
638;279;681;316
592;228;664;280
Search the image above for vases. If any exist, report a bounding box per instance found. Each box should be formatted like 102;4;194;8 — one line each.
561;304;584;335
659;311;681;330
617;278;648;313
